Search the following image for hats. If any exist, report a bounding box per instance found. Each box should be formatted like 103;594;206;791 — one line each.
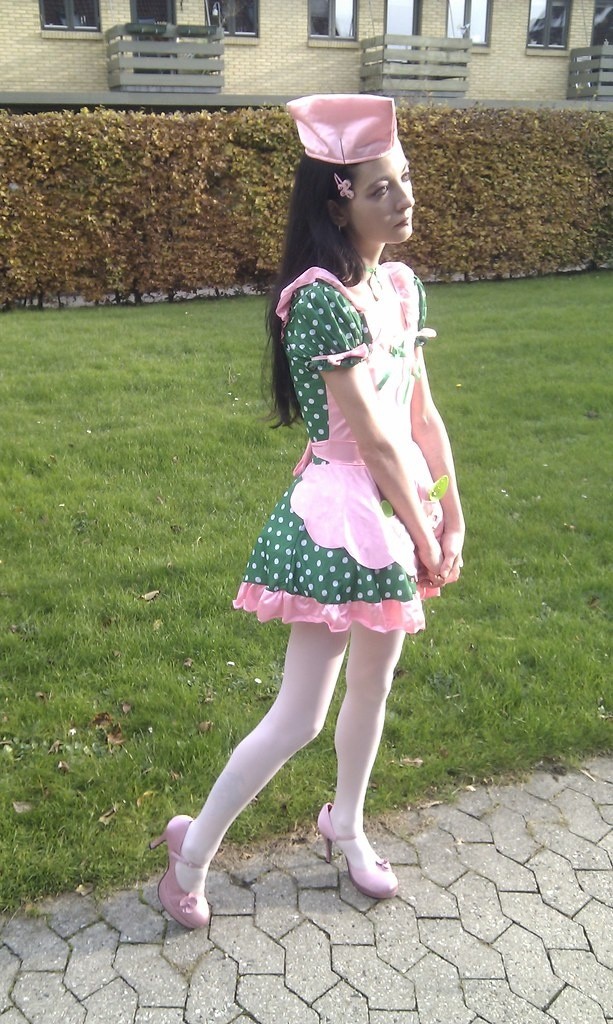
286;91;402;165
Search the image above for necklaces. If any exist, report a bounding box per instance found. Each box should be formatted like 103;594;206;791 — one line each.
359;257;385;302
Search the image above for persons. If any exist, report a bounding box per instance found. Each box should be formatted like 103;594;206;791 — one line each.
144;92;469;930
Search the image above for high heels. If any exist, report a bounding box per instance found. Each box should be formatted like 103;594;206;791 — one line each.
147;812;212;930
316;802;401;903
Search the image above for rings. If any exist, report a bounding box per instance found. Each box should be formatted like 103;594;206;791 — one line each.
431;573;443;582
